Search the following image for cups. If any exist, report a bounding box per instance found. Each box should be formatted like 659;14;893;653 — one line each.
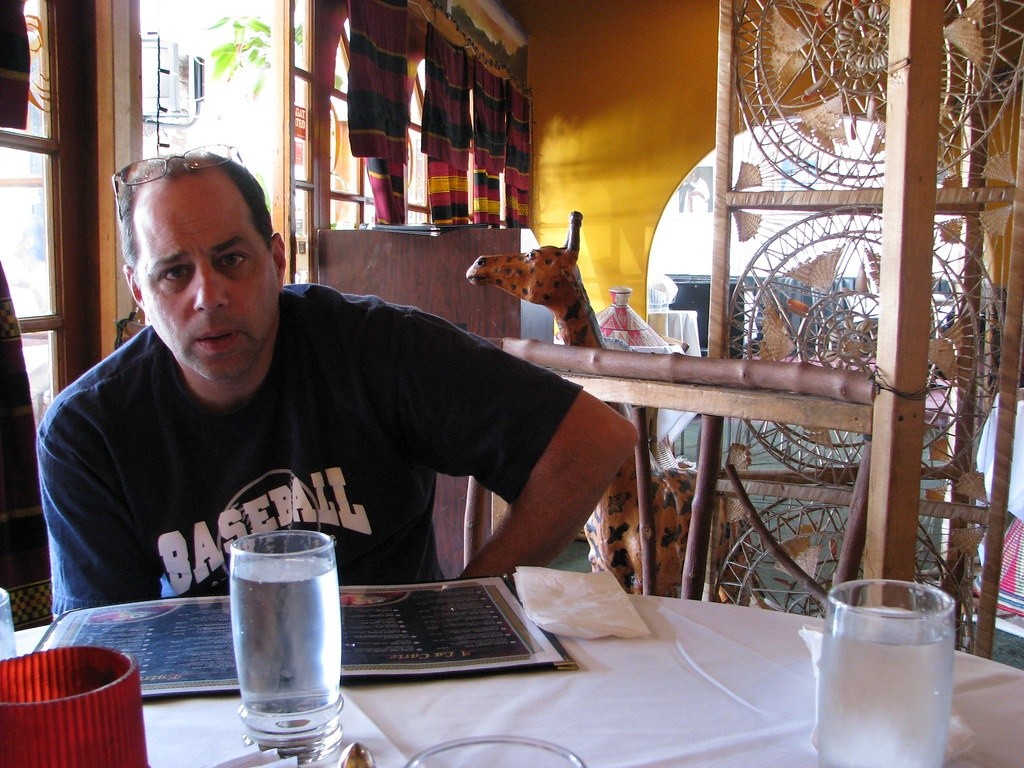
0;645;147;768
229;530;344;765
404;736;585;768
0;588;17;660
819;578;955;767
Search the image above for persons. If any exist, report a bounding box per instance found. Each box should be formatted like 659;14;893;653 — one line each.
679;168;710;213
36;144;640;629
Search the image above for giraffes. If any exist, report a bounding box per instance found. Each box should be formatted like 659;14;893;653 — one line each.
464;209;746;598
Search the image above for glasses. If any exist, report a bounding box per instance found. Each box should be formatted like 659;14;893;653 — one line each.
111;144;261;222
